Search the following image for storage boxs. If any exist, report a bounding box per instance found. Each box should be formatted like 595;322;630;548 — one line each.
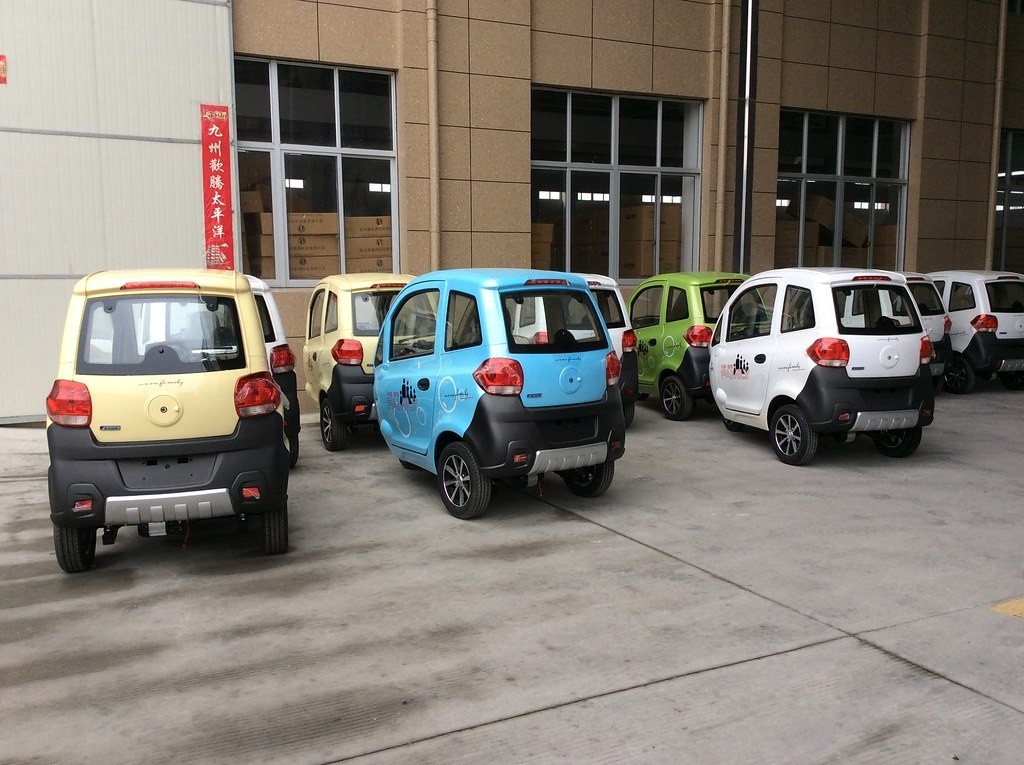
235;162;894;287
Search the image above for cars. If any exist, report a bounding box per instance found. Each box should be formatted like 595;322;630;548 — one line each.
706;266;936;467
301;271;454;453
785;272;954;395
893;269;1024;395
45;267;293;575
375;266;626;520
451;272;639;431
621;271;772;421
129;275;302;470
